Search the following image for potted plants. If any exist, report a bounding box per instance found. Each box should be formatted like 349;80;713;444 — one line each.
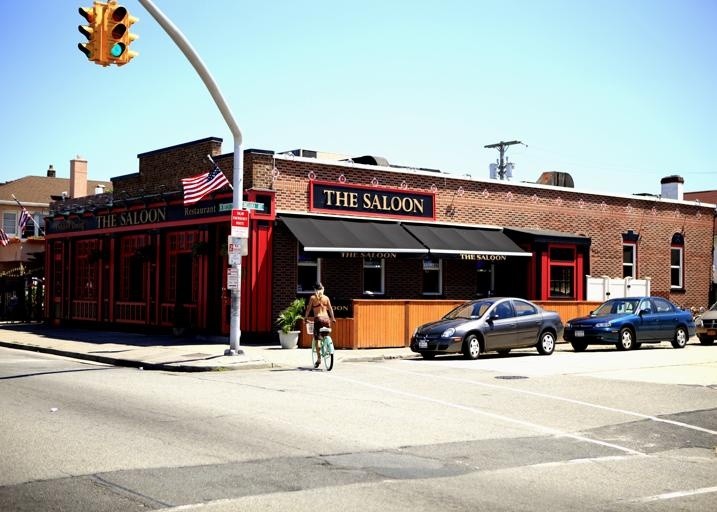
275;297;306;349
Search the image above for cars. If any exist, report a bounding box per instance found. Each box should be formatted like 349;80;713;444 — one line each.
563;296;696;351
409;296;564;360
696;300;717;345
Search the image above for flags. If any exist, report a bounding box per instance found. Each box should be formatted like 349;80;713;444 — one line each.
181;162;229;207
18;208;31;235
0;228;10;247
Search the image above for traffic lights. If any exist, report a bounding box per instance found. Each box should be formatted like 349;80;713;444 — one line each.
104;4;127;64
127;14;139;63
76;4;100;62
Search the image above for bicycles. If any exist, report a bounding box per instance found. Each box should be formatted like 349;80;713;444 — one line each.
303;319;337;371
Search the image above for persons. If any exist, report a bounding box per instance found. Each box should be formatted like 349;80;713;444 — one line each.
303;282;336;368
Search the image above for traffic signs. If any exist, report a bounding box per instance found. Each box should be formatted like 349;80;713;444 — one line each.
243;201;264;211
231;209;249;227
219;203;233;211
